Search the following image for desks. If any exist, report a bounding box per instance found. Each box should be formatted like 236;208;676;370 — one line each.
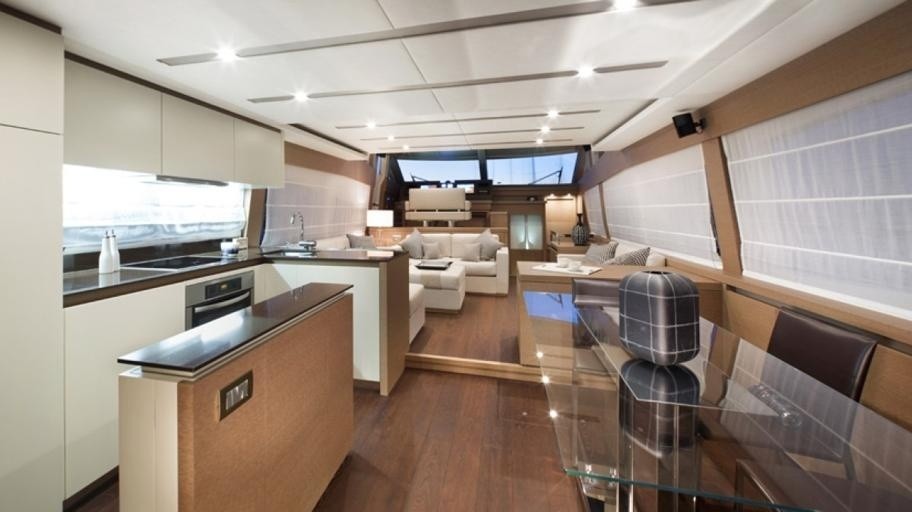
409;262;466;314
523;290;912;512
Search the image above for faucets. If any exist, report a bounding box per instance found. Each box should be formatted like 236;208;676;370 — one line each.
290;210;304;241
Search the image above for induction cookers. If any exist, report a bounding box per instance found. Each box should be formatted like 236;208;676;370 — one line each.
122;252;248;273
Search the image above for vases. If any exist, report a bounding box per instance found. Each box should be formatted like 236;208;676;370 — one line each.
572;213;588;246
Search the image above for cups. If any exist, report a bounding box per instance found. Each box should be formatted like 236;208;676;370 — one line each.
557;257;581;271
220;242;240;257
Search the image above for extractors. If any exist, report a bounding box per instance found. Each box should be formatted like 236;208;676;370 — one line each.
137;173;228;186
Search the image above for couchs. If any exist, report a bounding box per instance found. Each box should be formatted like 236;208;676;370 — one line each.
393;227;510;297
405;188;472;227
556;238;666;266
281;230;404;252
409;283;426;345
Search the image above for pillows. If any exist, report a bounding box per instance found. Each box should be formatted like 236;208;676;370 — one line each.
346;233;377;250
398;228;505;262
601;247;650;266
581;242;618;266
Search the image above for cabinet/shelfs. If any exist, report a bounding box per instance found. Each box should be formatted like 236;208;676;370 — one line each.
63;51;161;177
162;86;234;183
235;113;286;187
1;3;64;512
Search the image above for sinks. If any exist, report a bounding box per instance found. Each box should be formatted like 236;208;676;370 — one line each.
259;250;317;258
120;254;238;272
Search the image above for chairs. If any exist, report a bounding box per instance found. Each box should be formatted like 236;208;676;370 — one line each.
734;455;912;511
747;306;878;482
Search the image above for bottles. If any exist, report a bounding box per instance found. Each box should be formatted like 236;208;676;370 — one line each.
97;230;113;273
109;228;121;270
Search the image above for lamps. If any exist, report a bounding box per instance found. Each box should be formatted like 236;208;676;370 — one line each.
366;210;394;247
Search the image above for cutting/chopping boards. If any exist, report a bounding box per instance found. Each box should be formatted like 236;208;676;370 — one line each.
533;263;602;275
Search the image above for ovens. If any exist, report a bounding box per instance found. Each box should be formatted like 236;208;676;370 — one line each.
184;271;257;331
